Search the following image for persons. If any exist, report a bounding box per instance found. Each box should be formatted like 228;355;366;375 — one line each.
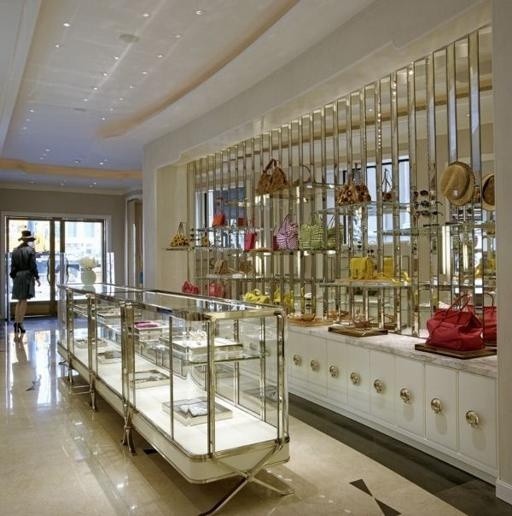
9;230;41;332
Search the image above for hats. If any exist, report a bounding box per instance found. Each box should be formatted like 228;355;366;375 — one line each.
18;230;36;242
439;161;474;205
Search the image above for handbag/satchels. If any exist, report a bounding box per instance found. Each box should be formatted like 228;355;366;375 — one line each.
275;293;295;314
171;233;190;246
350;258;374;279
210;283;224;297
212;215;225;226
427;309;476;352
483;308;498;340
383;258;396;278
258;169;286;191
214;260;226;274
182;281;198;294
358;184;371;202
274;223;326;250
245;292;269;305
335;186;357;205
328;224;344;249
244;233;256;249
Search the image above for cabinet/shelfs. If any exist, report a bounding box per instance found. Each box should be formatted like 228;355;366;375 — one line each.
348;318;371;429
310;318;326;406
163;179;427;290
288;321;310;401
371;331;397;441
48;282;293;510
326;320;348;416
221;318;288;390
458;353;497;488
397;334;424;454
424;339;460;466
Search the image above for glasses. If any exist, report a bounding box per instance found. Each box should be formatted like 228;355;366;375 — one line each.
411;191;432;218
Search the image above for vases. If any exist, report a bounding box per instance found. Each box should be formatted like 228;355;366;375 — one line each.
81;267;97;285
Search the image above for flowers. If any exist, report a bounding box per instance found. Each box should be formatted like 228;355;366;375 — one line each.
78;257;99;270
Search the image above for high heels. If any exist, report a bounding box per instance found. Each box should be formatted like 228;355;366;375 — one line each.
14;322;26;333
